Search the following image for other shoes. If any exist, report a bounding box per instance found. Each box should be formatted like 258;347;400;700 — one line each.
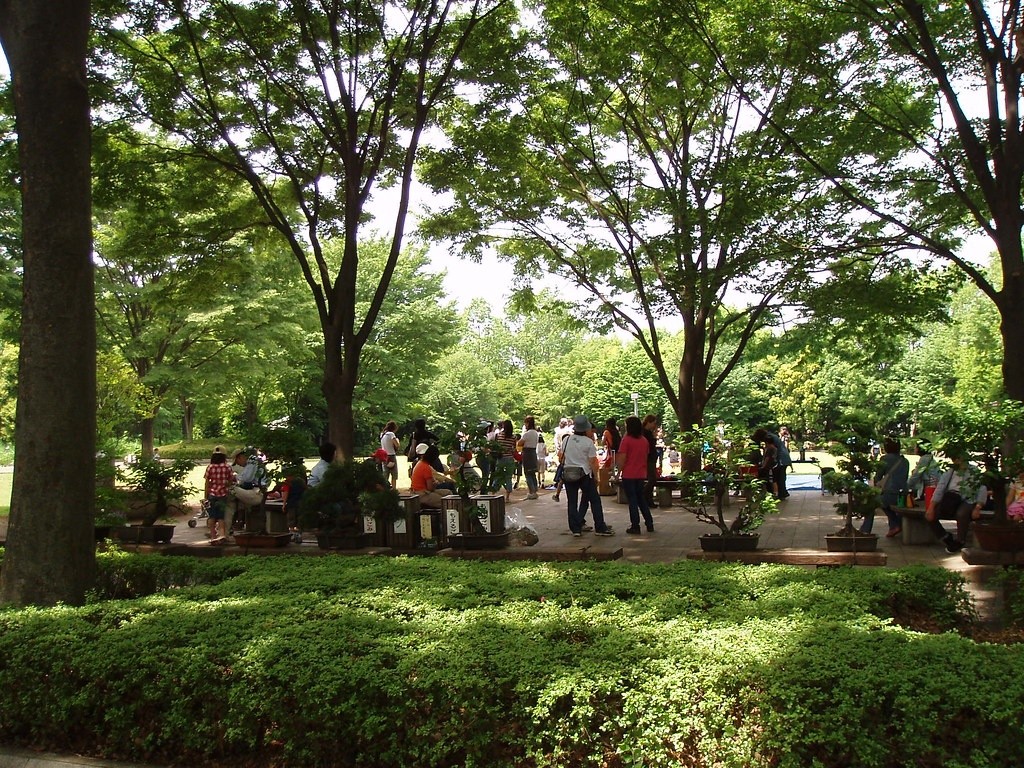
595;527;615;536
552;494;559;502
649;502;659;509
647;526;654;532
942;534;963;554
783;491;790;498
524;493;539;500
626;526;641;535
887;527;902;537
580;524;593;530
573;531;580;536
211;535;226;545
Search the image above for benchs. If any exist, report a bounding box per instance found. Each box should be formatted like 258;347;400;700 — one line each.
890;503;996;546
609;477;768;508
420;507;444;541
263;499;288;535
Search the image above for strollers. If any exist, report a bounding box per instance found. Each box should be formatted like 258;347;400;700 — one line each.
188;500;209;528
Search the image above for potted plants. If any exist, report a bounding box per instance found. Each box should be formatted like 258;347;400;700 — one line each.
93;454;129;541
666;423;782;552
129;447;198;545
444;413;511;549
929;393;1024;551
817;414;886;554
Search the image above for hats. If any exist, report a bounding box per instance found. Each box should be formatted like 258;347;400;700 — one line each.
416;443;429;455
371;449;388;462
213;444;227;456
573;414;592;432
232;448;243;467
479;421;492;430
458;450;472;461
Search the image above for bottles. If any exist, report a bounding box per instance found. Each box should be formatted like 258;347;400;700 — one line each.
897;489;906;508
907;489;915;508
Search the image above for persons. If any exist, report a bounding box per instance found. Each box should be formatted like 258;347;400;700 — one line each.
859;436;1024;554
735;427;794;500
405;419;481;543
153;448;160;459
380;421;400;489
307;444;336;487
203;445;237;539
475;418;549;502
552;418;600;531
643;414;659;507
668;445;680;467
600;419;622;470
223;449;270;540
655;428;667;475
702;421;724;459
617;416;655;534
365;448;388;493
561;414;615;536
281;464;308;543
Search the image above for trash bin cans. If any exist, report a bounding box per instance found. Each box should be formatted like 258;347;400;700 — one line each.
386;494;420;549
469;494;504;534
359;493;383;546
417;509;442;547
441;495;472;544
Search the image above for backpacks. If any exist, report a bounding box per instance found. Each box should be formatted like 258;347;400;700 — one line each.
605;428;621;451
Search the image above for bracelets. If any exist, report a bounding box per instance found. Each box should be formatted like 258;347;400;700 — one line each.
977;504;981;509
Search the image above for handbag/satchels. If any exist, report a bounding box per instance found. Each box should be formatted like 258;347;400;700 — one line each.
407;432;416;460
239;481;254;490
877;475;889;489
564;466;584;482
554;464;563;482
940;489;961;517
